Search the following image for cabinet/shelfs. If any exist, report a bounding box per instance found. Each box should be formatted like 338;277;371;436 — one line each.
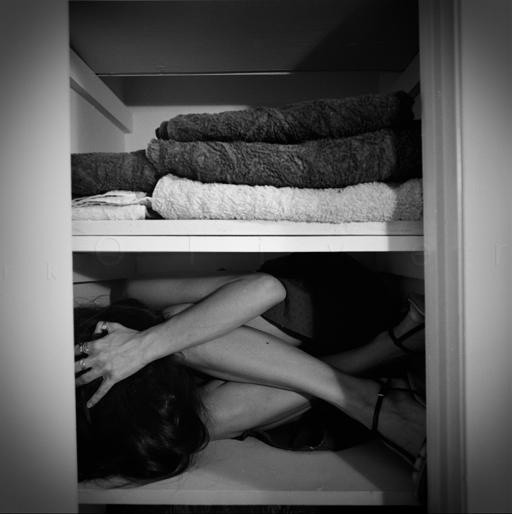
0;2;509;512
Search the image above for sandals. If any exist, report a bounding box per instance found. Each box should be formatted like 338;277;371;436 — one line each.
388;292;425;355
357;365;427;503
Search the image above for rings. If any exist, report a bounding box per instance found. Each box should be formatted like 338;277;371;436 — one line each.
79;357;86;370
78;341;89;353
101;321;108;330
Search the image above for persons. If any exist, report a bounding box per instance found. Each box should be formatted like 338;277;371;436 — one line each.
73;269;429;500
